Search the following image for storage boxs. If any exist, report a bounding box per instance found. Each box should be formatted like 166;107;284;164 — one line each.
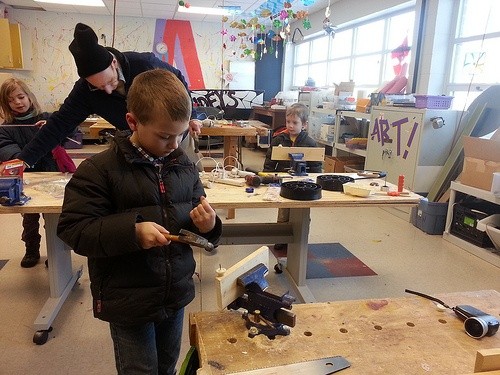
324;156;365;173
410;199;449;235
462;135;500;191
344;165;364;173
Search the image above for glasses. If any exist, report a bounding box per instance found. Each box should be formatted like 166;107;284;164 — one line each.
87;63;117;91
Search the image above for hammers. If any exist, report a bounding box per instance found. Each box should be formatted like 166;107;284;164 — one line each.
162;229;214;252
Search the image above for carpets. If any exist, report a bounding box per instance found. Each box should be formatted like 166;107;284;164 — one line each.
268;243;378;279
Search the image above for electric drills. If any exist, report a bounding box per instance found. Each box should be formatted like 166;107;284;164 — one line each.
245;173;293;187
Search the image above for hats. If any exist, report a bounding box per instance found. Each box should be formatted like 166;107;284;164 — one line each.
68;22;113;79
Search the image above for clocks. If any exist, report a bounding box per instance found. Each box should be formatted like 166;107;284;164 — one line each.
156;42;168;54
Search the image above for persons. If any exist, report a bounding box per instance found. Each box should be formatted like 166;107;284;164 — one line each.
261;104;323;250
55;68;222;375
15;22;205;173
0;78;65;269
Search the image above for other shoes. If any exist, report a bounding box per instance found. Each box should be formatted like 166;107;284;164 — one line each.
21;244;40;267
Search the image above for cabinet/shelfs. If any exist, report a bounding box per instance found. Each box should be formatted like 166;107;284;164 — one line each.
443;181;500;267
365;106;466;198
309;108;371;157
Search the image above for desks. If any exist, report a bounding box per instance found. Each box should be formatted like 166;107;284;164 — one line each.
189;291;500;375
66;117;270;171
253;106;286;130
0;172;428;344
